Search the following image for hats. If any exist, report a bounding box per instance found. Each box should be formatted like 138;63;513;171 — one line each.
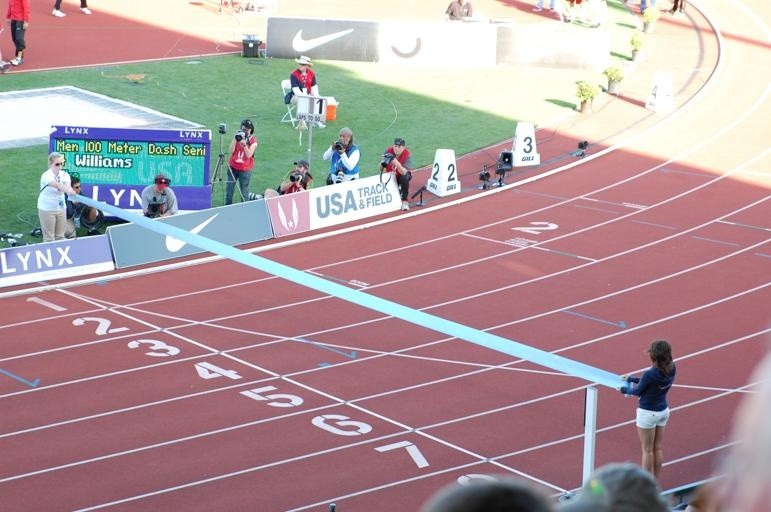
295;56;313;67
294;160;309;169
156;174;166;189
555;463;674;512
394;139;405;146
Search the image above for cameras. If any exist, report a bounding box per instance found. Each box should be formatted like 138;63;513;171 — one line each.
234;128;247;142
218;123;225;134
210;154;245;202
499;150;513;172
290;172;308;184
478;171;490;182
379;152;395;168
333;141;344;151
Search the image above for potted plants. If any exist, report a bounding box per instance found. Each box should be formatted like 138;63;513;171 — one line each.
630;30;643;62
642;6;660;33
577;80;595;113
602;65;624;97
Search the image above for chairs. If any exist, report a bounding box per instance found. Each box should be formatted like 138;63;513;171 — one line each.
281;80;316;127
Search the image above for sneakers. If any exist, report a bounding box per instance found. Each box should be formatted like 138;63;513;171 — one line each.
0;57;24;70
80;8;92;15
88;230;100;235
51;8;66;17
402;203;410;211
313;121;327;128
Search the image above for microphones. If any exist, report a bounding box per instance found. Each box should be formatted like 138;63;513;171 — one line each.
411;185;428;200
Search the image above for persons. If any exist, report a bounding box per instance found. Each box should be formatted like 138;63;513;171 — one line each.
50;1;92;18
322;126;362;186
379;137;414;212
686;352;771;511
283;55;327;130
264;159;313;199
141;173;178;220
444;0;472;22
616;339;676;477
225;119;258;204
36;153;76;243
5;0;31;67
65;175;102;237
